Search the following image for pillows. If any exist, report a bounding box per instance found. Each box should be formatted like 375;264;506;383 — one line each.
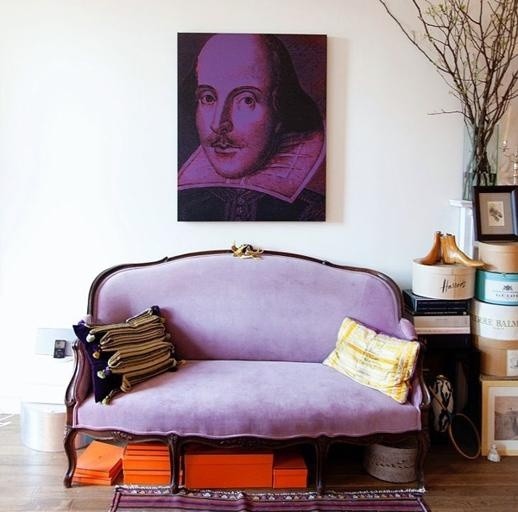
69;304;186;404
321;309;421;401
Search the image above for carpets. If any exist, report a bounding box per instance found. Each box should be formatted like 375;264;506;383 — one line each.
104;480;433;510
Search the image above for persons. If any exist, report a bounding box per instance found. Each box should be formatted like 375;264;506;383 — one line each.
178;34;324;221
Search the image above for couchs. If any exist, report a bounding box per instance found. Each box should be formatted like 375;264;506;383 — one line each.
62;243;434;494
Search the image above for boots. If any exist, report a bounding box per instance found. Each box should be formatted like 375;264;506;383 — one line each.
440;234;484;268
417;231;443;264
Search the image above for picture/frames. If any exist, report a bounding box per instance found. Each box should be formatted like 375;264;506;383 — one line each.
478;378;518;462
472;185;517;242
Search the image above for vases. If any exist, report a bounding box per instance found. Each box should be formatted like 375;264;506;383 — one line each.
462;120;498;200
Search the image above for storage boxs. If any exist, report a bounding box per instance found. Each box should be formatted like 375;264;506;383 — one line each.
266;448;314;489
410;260;473;301
468;240;517;380
173;443;272;495
399;289;469;318
412;313;470;336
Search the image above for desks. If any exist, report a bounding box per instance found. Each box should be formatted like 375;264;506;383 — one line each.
410;330;481;458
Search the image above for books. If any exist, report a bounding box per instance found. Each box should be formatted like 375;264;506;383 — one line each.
70;440;175;486
403;310;471;327
414;327;471;334
